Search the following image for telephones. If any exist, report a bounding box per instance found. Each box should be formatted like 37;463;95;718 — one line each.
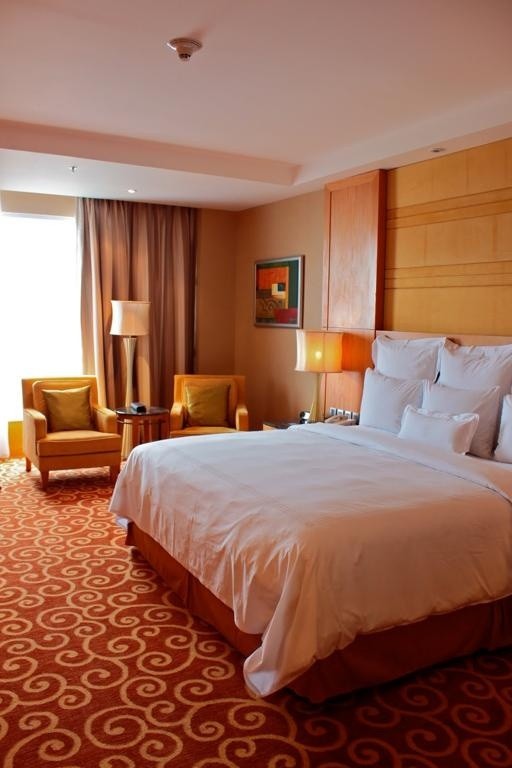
324;414;357;425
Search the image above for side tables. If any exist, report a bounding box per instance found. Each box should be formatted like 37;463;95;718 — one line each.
105;402;170;473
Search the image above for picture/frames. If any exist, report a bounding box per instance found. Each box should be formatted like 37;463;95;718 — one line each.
250;253;308;332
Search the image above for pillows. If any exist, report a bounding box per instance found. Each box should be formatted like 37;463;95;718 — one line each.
181;382;234;429
41;383;96;433
355;328;512;465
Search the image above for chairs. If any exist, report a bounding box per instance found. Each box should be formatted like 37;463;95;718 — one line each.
18;373;119;491
166;371;250;438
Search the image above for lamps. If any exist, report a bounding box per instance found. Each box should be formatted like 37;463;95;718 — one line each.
291;326;345;419
107;295;152;408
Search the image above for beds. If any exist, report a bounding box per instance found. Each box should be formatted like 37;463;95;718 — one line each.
109;325;511;707
